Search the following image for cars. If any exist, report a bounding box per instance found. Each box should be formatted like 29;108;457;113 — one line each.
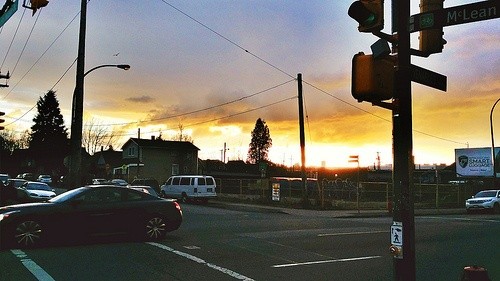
466;190;500;212
17;182;56;203
0;185;184;248
90;178;160;194
0;173;35;194
37;175;53;184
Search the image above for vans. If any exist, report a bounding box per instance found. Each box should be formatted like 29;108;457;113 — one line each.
161;175;217;203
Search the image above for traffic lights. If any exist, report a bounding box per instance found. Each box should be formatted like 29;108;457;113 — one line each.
348;0;384;33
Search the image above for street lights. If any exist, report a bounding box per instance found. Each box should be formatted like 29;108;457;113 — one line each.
70;64;130;192
